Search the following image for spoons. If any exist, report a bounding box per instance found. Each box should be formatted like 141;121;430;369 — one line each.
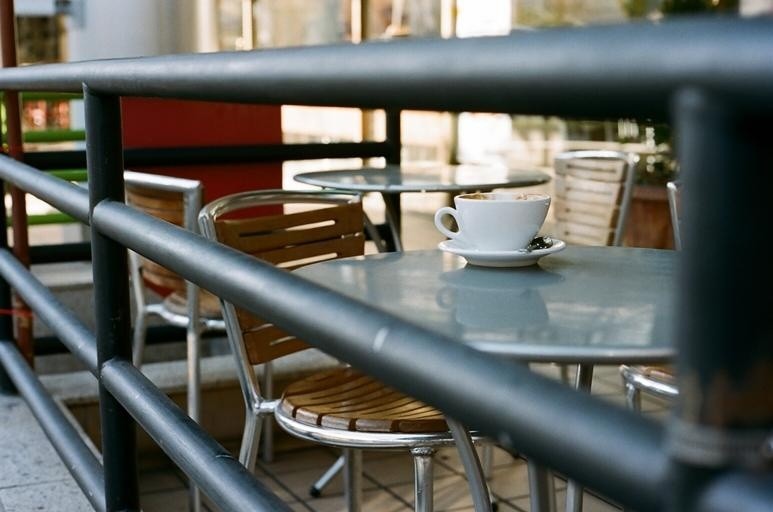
526;235;554;254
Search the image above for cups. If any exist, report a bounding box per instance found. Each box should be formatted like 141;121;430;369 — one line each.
434;192;551;252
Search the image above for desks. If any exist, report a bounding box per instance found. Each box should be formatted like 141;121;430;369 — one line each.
294;164;550;252
287;243;681;511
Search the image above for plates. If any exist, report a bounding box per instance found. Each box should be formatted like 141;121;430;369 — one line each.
437;238;564;268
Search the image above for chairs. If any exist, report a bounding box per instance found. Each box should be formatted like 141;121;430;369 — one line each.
122;170;228;426
197;190;488;510
551;151;635;248
